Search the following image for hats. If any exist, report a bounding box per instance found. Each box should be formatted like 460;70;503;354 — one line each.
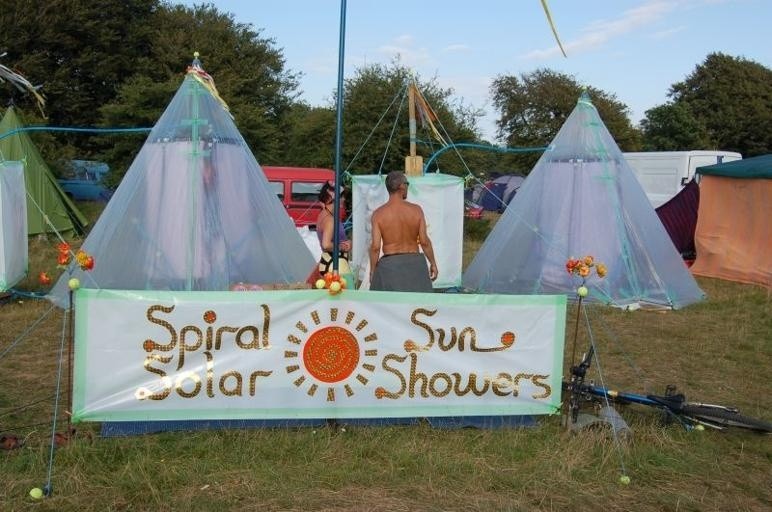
319;179;345;205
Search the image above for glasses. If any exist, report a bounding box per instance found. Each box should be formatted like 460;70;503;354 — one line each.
399;181;410;188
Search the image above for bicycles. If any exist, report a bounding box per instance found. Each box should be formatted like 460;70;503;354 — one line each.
563;346;771;437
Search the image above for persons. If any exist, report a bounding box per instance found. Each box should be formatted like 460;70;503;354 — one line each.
315;179;351;277
367;170;438;292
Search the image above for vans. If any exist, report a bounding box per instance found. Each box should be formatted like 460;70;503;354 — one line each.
261;166;348;233
53;160;113;202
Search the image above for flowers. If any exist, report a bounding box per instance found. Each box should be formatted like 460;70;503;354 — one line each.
566;256;608;298
314;270;345;296
37;242;95;291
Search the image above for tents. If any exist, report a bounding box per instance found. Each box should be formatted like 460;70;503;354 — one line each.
0;104;92;238
687;150;772;289
464;172;527;215
45;60;319;313
460;83;708;313
